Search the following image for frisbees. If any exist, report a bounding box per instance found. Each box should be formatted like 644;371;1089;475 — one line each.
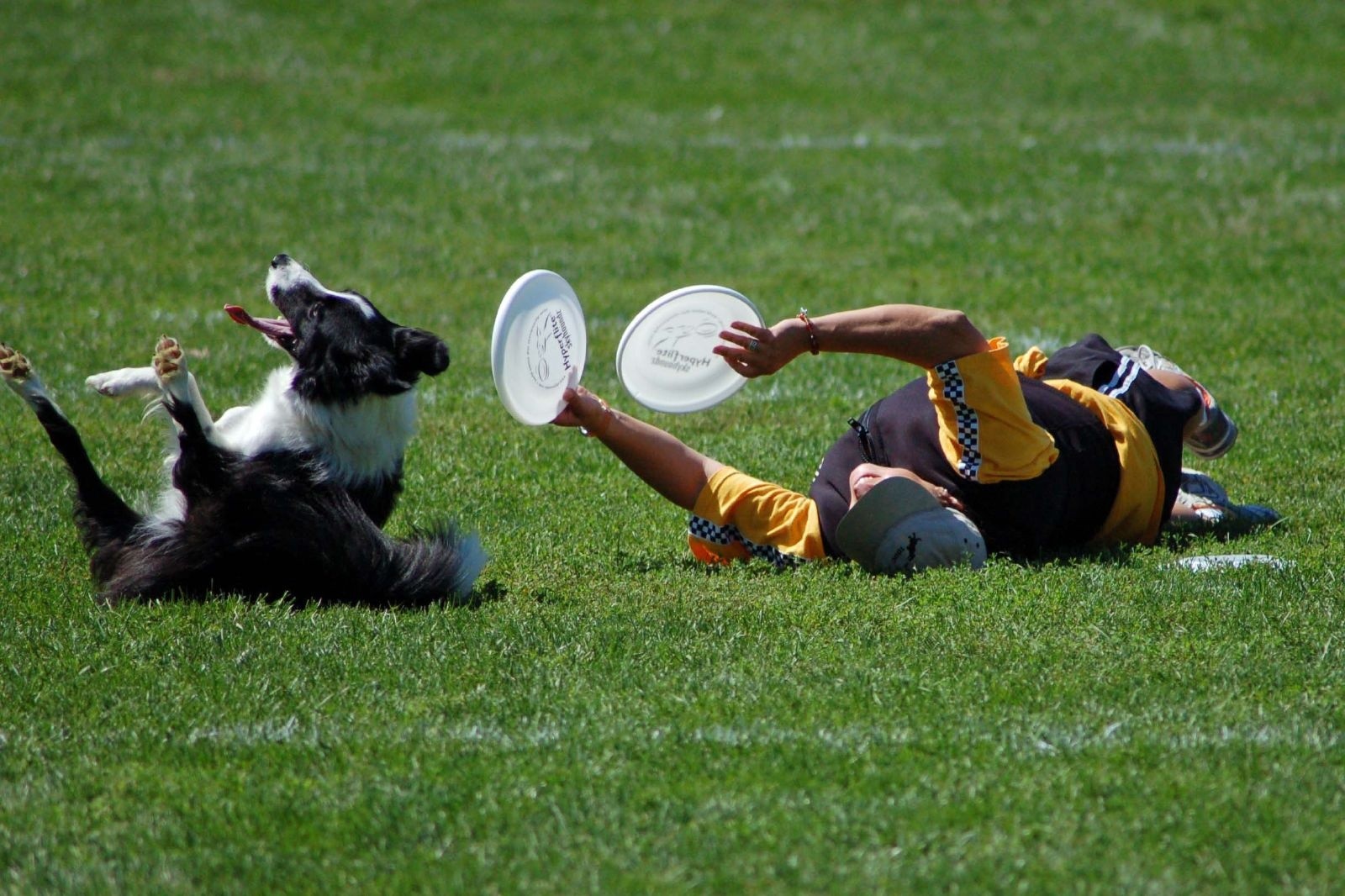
491;270;589;426
616;285;767;415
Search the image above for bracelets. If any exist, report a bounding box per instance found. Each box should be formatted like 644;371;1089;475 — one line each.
796;307;819;355
577;399;610;437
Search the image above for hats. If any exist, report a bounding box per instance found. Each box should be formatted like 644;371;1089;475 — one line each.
836;477;987;578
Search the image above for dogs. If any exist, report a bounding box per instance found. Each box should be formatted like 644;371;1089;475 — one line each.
0;249;492;613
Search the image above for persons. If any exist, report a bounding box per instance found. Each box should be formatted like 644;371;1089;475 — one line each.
548;304;1282;580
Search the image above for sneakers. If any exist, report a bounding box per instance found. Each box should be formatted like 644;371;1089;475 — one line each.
1113;344;1238;459
1179;468;1282;537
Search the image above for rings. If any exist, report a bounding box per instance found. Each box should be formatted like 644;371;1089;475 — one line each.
745;339;758;351
